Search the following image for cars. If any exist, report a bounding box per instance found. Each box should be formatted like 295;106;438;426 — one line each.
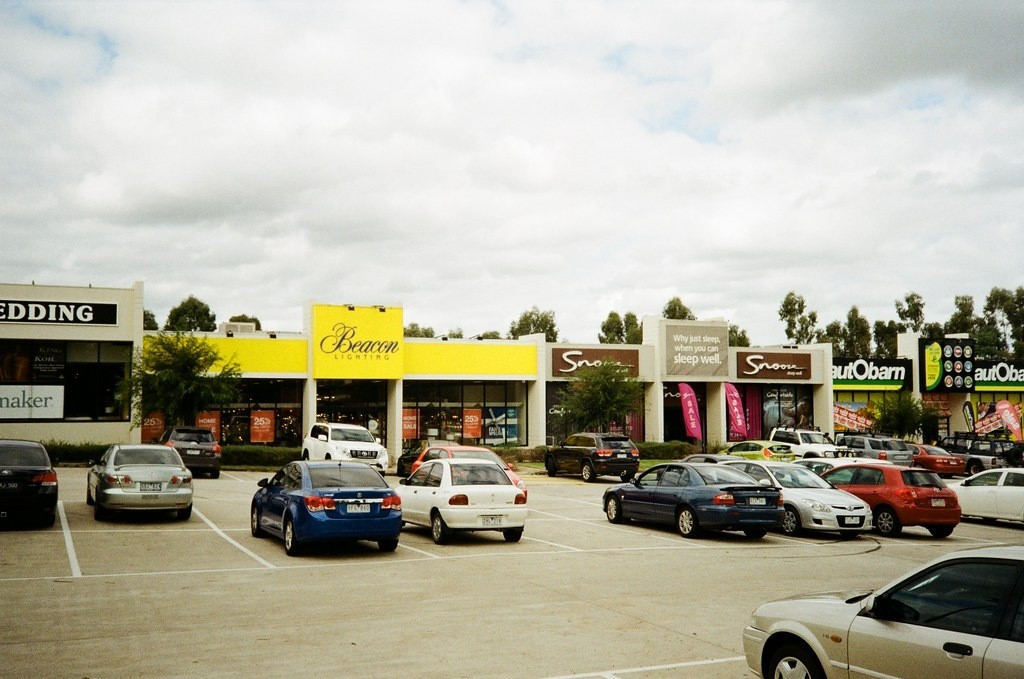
410;446;528;497
717;440;795;462
947;468;1024;522
397;440;461;478
87;443;194;522
906;444;965;479
0;439;59;528
717;461;873;539
741;541;1023;678
819;463;960;539
657;454;750;476
601;463;786;539
395;458;527;544
252;459;404;557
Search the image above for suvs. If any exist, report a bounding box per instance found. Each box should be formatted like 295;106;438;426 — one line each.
769;425;859;460
153;427;221;478
938;431;1016;476
832;433;913;467
301;421;388;480
230;416;249;435
544;433;640;483
